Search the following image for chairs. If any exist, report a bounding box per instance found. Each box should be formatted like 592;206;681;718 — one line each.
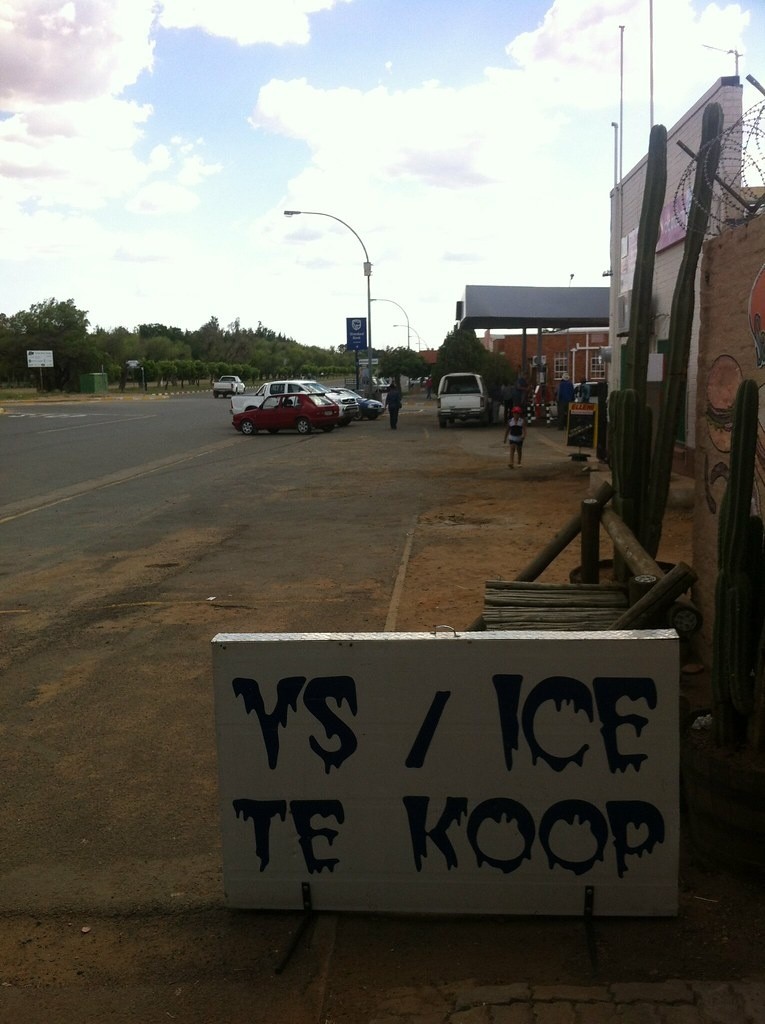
284;400;294;408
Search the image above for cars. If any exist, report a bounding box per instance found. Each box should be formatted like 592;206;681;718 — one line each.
371;376;390;392
231;392;340;434
330;387;385;421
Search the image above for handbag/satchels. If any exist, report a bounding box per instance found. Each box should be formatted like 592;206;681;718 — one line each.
399;403;402;409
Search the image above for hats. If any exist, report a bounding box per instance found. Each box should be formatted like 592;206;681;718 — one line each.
562;373;569;380
511;406;521;413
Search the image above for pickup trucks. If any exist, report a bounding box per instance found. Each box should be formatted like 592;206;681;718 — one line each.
229;380;360;429
437;373;494;429
213;376;246;398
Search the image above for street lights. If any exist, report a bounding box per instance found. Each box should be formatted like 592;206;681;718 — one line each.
284;210;373;399
370;298;421;353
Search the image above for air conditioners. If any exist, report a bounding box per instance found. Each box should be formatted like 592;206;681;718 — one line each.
533;356;547;366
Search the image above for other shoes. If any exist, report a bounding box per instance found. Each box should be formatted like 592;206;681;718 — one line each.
508;464;514;469
517;465;522;468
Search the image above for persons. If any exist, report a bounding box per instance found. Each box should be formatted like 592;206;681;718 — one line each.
385;384;402;430
504;406;527;468
557;373;590;430
487;373;531;424
425;377;432;400
